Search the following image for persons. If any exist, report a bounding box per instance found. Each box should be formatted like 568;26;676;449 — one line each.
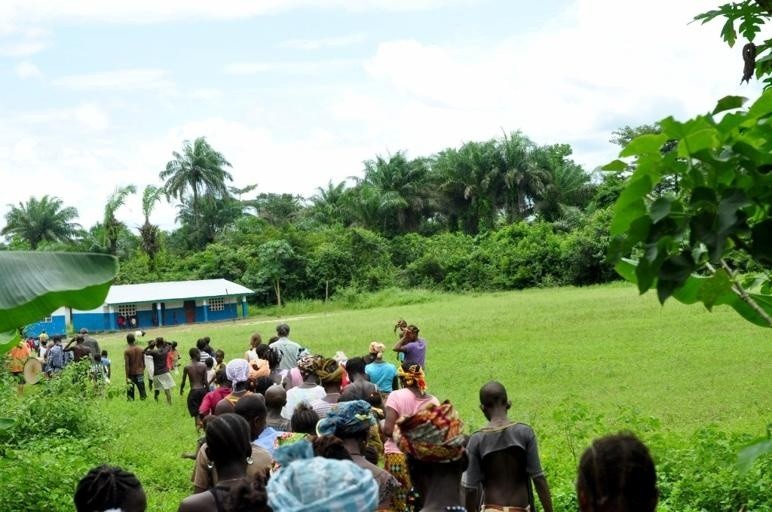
73;464;148;511
124;332;181;407
177;316;660;511
5;325;111;393
